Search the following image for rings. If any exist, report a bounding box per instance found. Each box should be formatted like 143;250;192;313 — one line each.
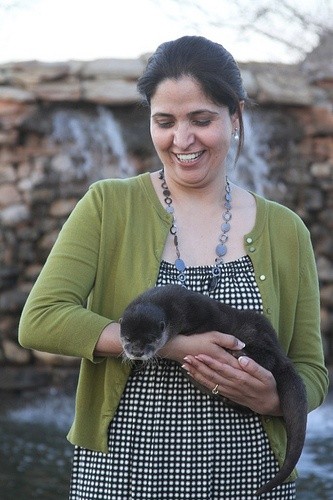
211;384;219;394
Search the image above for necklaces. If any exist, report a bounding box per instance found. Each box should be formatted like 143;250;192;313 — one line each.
158;168;231;295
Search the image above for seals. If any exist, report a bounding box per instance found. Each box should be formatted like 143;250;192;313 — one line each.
115;283;310;498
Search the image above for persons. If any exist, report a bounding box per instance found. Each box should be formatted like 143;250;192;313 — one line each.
18;35;329;500
